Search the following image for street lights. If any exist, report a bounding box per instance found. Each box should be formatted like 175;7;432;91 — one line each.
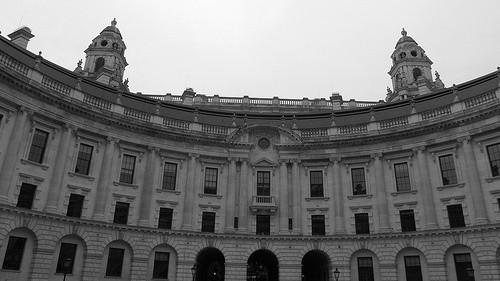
61;257;72;281
466;265;474;281
333;267;341;281
190;263;198;281
